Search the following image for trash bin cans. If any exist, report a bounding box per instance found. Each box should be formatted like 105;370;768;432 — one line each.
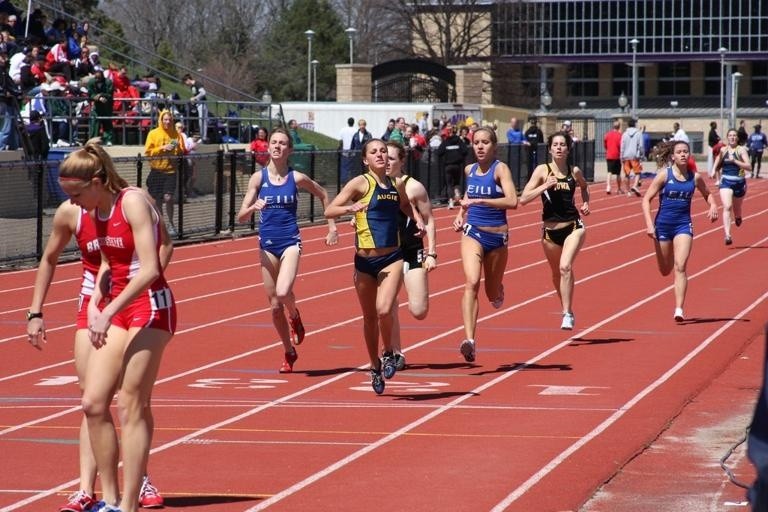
46;148;80;207
339;152;364;194
641;132;648;162
287;143;315;193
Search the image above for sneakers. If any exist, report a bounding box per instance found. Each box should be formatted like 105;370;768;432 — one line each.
674;308;685;323
447;200;456;209
725;237;732;245
138;476;165;509
491;283;506;310
616;186;642;198
279;347;297;374
288;307;305;345
561;312;575;331
369;349;405;394
715;181;720;186
460;340;476;363
606;185;612;194
167;226;178;237
734;216;742;227
59;492;123;512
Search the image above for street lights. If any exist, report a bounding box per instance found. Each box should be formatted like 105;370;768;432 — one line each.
344;26;358;65
630;38;642;124
538;87;556;115
718;46;728;147
259;87;274;105
616;89;631;114
731;69;745;132
312;58;320;103
304;28;314;103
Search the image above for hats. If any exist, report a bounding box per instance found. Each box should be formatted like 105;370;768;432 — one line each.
562;120;573;126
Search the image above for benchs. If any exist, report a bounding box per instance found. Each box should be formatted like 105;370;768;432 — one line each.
78;110;239;144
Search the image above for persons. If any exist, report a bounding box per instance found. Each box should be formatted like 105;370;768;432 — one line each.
0;0;768;511
